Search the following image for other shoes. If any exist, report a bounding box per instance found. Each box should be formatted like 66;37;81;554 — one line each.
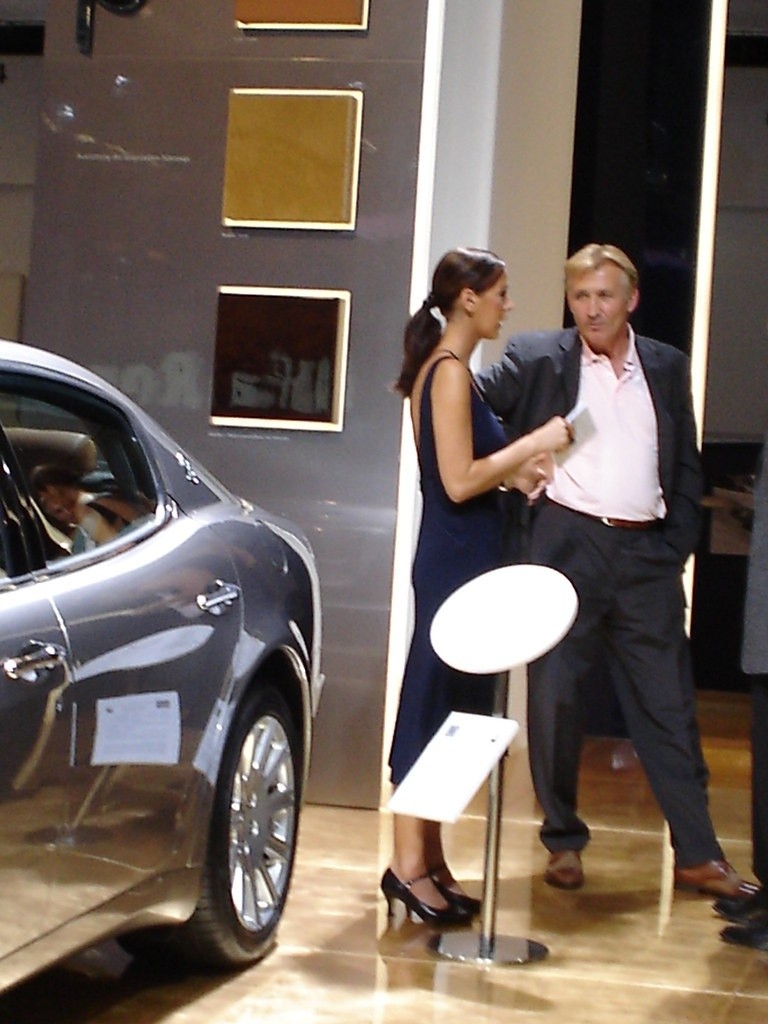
713;901;768;923
719;914;768;951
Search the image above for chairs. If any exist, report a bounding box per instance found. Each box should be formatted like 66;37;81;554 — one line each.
6;426;97;554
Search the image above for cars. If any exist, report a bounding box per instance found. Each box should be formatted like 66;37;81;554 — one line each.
0;339;325;995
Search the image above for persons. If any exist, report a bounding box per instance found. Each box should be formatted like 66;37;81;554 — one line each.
474;243;760;898
29;461;142;549
381;249;574;927
712;449;768;947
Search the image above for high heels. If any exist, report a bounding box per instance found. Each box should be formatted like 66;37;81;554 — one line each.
381;867;473;927
406;863;481;916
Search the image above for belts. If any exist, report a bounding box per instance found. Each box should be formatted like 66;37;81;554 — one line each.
544;496;658;529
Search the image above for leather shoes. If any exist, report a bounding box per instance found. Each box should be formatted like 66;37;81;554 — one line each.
544;850;583;888
673;859;759;900
